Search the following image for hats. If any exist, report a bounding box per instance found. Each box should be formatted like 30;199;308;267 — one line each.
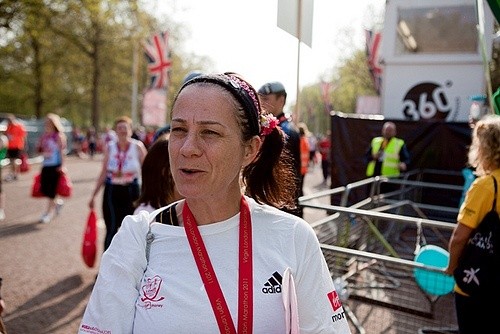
46;113;60;130
258;81;283;94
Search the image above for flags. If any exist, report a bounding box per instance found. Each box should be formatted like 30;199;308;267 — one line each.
366;31;386;95
319;76;338;114
140;32;172;90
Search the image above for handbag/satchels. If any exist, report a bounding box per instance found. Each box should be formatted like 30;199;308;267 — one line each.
57;170;72;196
32;173;47;198
82;209;97;267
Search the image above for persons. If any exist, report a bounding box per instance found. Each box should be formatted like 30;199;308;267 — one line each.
37;111;67;224
87;114;149;285
317;138;332;184
362;122;408;235
293;122;317;196
258;81;304;221
74;72;354;334
66;116;175;161
133;140;185;211
442;112;500;334
4;114;27;183
0;151;9;222
